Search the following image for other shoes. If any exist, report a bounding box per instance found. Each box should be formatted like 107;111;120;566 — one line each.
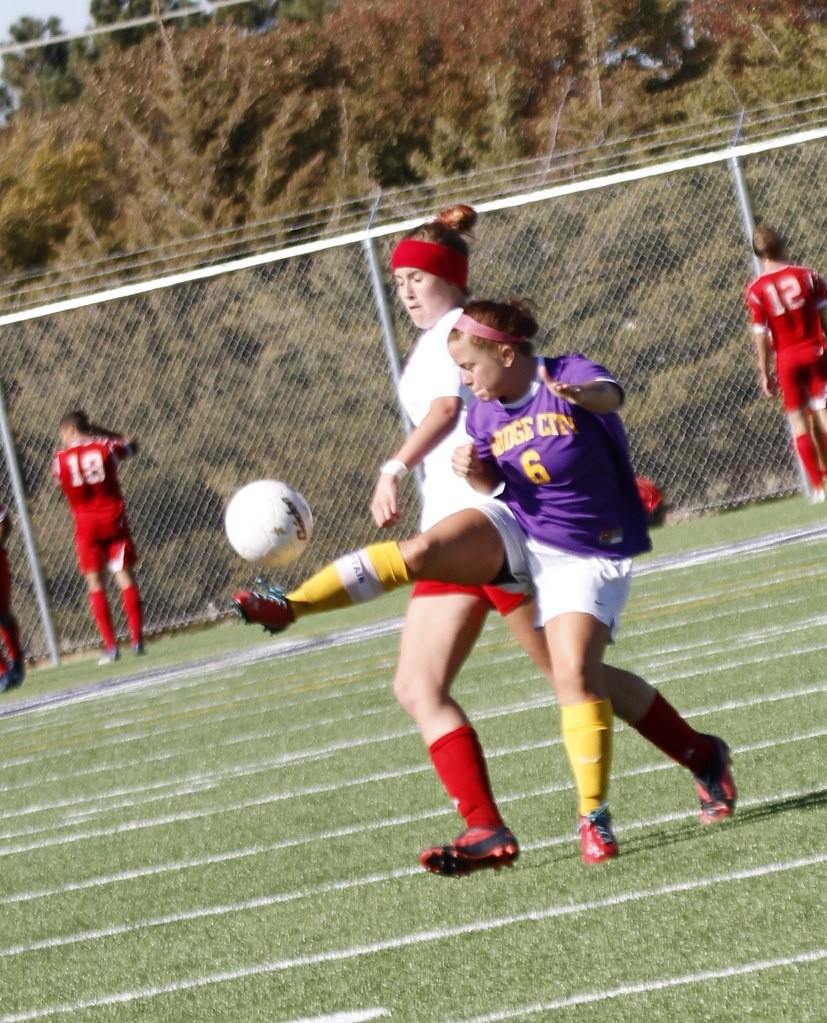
95;648;119;668
130;643;143;657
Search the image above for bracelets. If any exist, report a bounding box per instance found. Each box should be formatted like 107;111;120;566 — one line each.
380;460;410;482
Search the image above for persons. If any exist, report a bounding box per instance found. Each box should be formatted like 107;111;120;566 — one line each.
367;205;739;880
52;410;142;666
747;226;827;506
0;502;26;693
230;298;654;864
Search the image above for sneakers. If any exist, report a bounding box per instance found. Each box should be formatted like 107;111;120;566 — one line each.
419;825;518;878
694;734;737;824
578;803;618;862
230;591;295;636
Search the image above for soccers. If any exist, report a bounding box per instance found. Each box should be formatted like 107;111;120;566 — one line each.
224;479;313;568
